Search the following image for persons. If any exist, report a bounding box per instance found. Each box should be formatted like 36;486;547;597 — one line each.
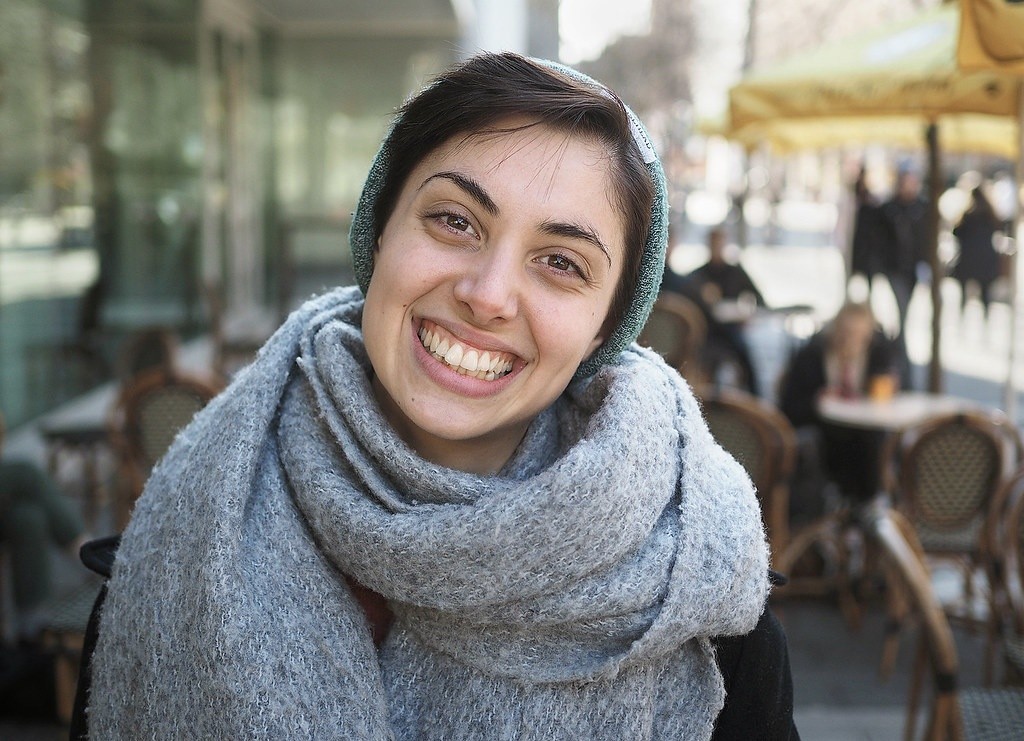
777;302;913;578
682;225;769;396
867;170;929;332
840;173;877;296
944;188;1001;321
67;49;801;741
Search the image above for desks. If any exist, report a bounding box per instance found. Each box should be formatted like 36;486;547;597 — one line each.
42;375;124;542
814;387;995;605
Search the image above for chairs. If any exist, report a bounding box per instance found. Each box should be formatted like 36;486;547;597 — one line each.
0;263;1024;741
